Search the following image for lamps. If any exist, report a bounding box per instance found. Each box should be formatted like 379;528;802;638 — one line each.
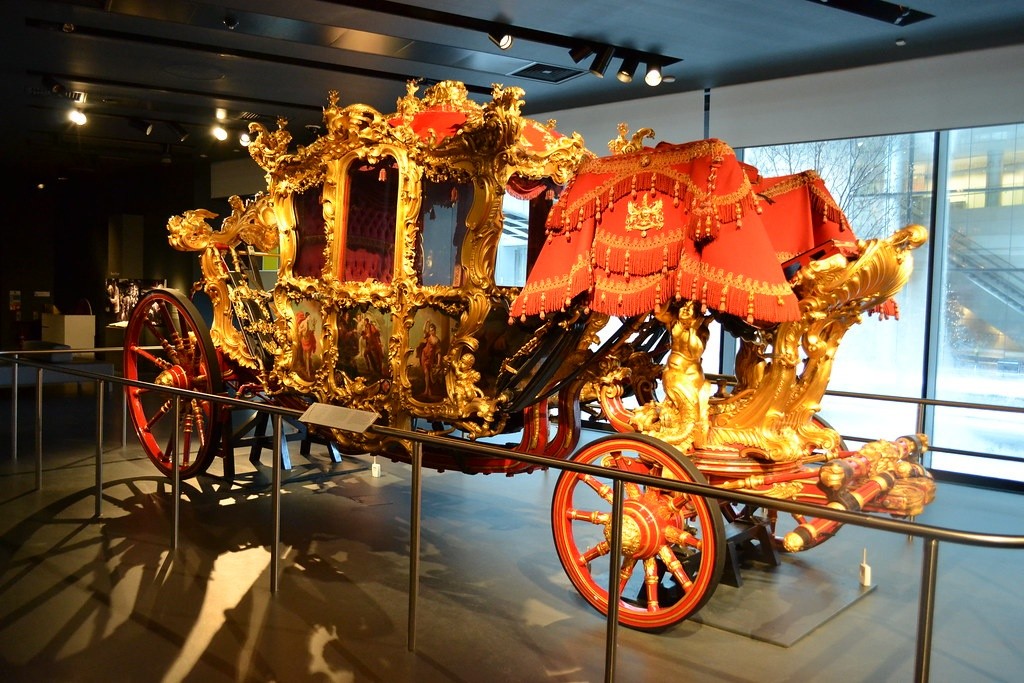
173;124;189;142
128;118;153;136
487;24;514;50
568;42;594;64
616;55;641;83
41;75;61;94
589;42;616;78
644;60;662;88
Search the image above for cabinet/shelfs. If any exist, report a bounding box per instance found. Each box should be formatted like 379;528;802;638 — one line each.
104;325;163;370
41;312;96;362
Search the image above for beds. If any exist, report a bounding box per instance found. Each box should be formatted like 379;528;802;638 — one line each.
0;349;114;392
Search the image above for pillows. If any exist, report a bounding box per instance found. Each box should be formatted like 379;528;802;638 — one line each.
23;341;72;363
